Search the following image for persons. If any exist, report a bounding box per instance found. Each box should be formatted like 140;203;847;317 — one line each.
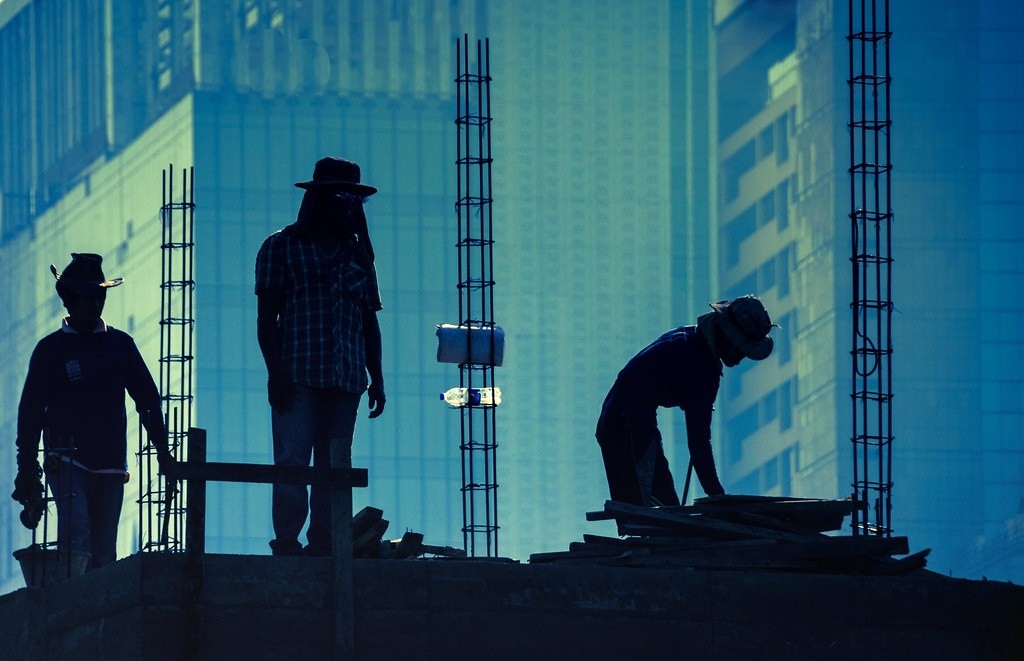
256;157;387;556
594;296;773;537
12;253;176;573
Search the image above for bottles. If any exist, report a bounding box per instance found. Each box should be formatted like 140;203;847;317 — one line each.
440;387;502;407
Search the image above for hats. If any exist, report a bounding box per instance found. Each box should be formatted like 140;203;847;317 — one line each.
294;157;378;198
50;253;124;289
709;295;773;361
